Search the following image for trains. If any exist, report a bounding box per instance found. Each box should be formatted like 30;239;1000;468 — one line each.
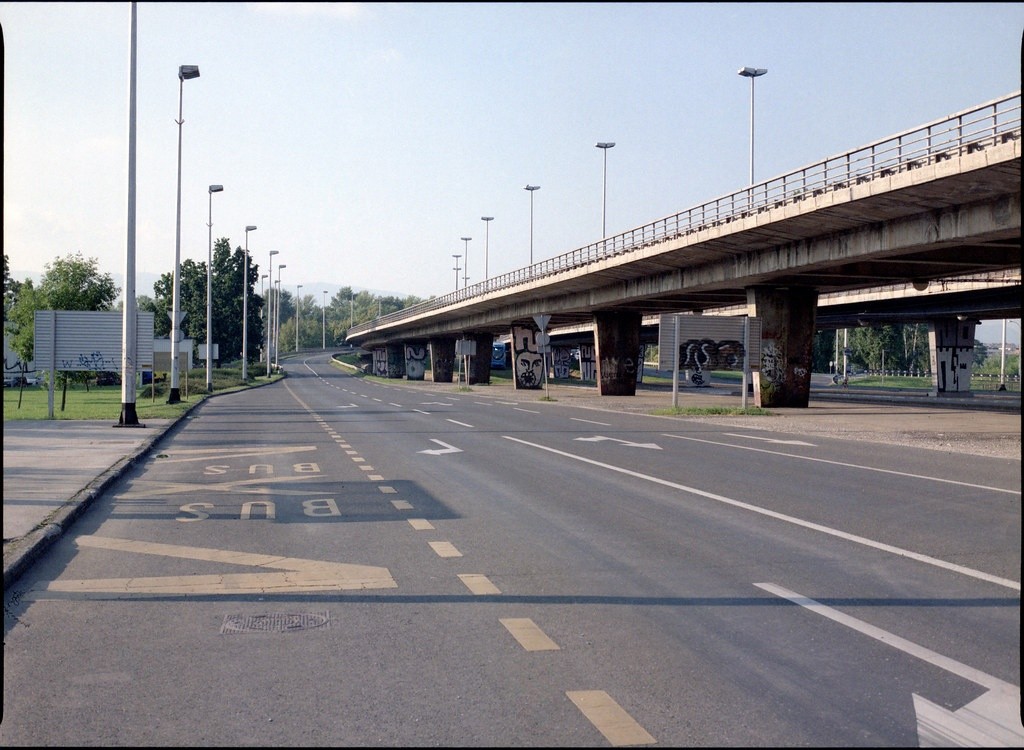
489;341;506;370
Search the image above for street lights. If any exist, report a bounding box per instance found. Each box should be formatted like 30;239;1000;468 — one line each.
377;295;384;318
206;185;224;394
737;66;768;208
267;250;280;378
296;284;303;352
166;65;201;404
593;142;615;257
275;265;287;370
259;274;268;361
323;290;328;349
351;292;356;348
481;217;496;292
452;254;462;300
273;279;281;348
453;267;462;300
1007;320;1021;375
522;184;542;277
461;236;473;298
241;225;258;381
461;276;470;298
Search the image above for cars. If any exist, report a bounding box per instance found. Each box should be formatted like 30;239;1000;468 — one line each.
96;372;123;386
4;372;45;388
